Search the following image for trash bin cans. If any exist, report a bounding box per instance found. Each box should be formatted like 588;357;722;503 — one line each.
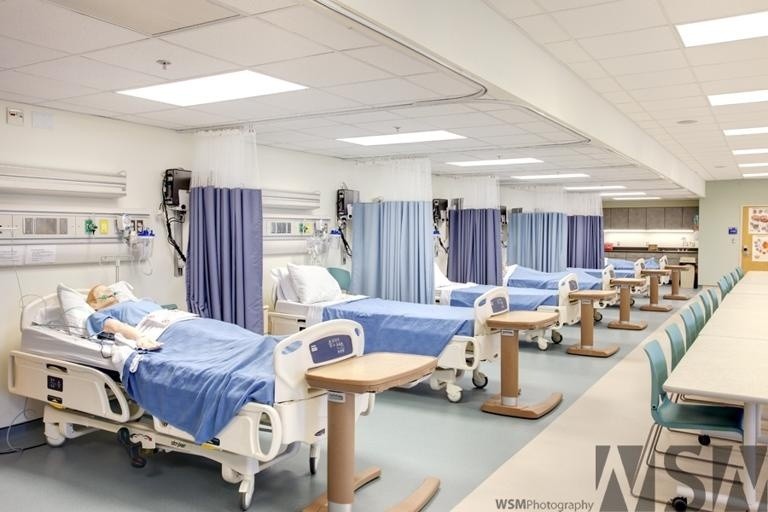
680;256;696;288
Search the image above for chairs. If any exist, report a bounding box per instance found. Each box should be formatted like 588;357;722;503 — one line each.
631;266;745;512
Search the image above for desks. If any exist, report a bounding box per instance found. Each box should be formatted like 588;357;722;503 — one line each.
663;262;690;300
662;270;768;512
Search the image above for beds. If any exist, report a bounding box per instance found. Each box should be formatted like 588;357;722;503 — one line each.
7;255;670;511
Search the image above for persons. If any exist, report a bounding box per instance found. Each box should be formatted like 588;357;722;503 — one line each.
85;282;169;351
